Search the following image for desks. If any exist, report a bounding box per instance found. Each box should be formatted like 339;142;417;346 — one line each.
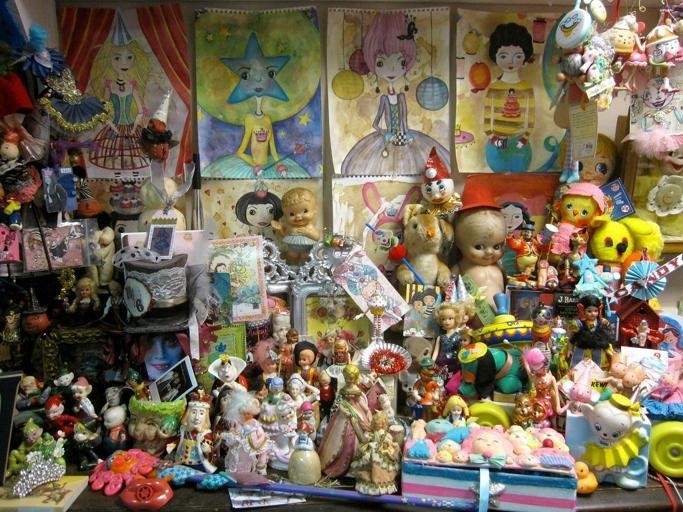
0;464;683;512
